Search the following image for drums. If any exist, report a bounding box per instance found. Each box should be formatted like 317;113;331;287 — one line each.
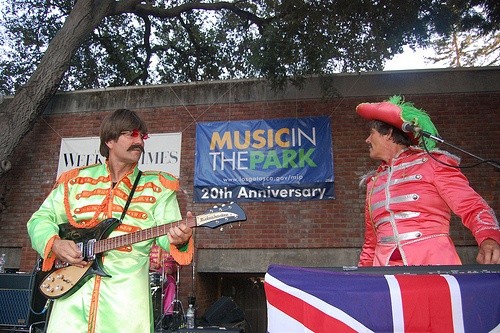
148;272;162;290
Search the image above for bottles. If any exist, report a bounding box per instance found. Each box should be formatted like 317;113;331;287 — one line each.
0;254;7;274
187;304;194;329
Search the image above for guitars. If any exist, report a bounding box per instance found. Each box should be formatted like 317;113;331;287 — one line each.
36;202;248;298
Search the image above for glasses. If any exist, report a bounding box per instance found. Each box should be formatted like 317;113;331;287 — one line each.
121;129;150;139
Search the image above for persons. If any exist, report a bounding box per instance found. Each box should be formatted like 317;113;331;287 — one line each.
356;95;500;267
27;109;194;333
149;243;182;331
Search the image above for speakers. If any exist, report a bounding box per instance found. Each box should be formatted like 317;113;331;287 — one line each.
0;274;48;330
202;295;244;326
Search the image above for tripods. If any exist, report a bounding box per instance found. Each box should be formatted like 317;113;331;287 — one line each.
154;263;187;331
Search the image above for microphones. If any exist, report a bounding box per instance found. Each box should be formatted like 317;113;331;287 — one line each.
401;122;421;134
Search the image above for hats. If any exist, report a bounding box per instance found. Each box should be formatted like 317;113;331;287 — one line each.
357;92;422;146
